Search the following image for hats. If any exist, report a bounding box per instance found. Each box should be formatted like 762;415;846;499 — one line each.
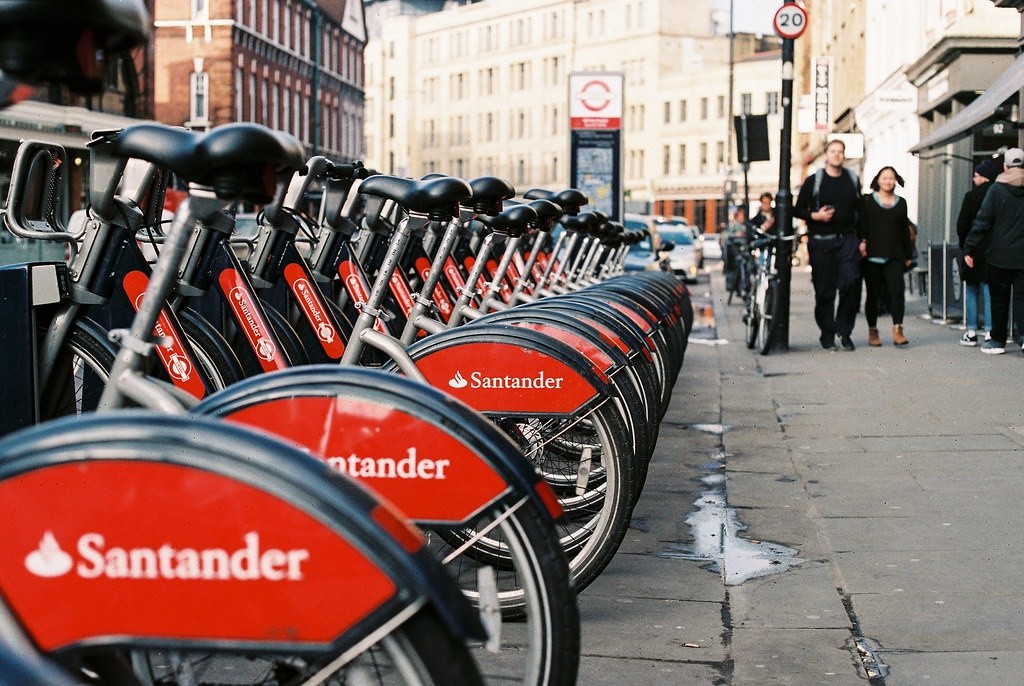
975;159;1000;180
1005;148;1024;167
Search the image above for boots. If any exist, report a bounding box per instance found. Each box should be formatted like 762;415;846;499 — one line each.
893;326;908;345
868;328;881;346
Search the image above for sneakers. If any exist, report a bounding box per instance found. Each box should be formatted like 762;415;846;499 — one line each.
983;336;993;342
959;331;977;346
980;338;1006;354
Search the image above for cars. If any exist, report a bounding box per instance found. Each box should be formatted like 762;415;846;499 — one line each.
656;224;705;284
666;217;689;225
623;211;675;282
691;223;707;270
644;214;668;226
701;233;724;259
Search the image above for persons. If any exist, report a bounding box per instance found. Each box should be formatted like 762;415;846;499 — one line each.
794;140;863;351
955;147;1023;355
903;216;918;273
861;166;913;346
716;193;774;276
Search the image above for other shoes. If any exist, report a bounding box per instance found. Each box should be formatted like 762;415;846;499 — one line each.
837;332;854;351
819;325;835;349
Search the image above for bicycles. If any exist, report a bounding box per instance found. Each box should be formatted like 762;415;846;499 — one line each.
2;1;695;686
720;219;809;353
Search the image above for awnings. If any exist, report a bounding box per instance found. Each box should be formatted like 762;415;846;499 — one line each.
907;52;1024;164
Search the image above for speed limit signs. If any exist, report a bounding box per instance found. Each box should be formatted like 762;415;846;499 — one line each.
772;4;808;39
